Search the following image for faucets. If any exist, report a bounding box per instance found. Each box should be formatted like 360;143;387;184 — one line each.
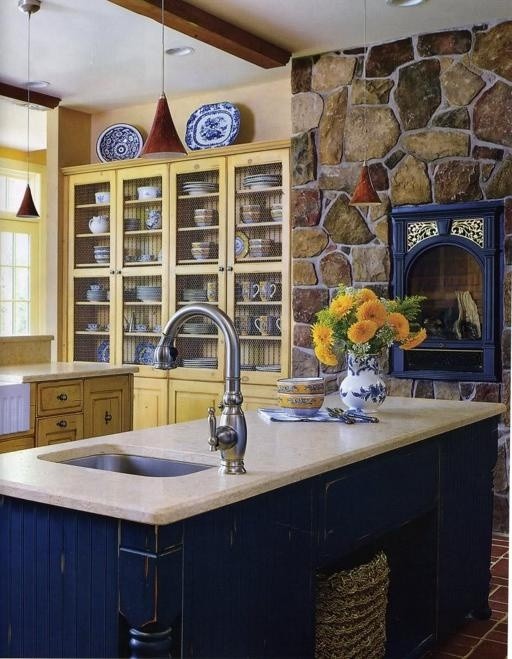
152;300;249;477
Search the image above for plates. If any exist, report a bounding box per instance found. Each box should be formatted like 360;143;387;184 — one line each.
181;181;216;194
242;173;281;187
96;122;143;163
119;217;141;231
180;288;216;368
240;364;281;372
86;286;161;302
184;101;241;150
98;340;110;363
134;342;154;365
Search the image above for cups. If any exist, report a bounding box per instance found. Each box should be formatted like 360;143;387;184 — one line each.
235;282;281;340
206;283;217;302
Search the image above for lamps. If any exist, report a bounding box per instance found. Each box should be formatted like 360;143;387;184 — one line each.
348;1;383;207
17;0;40;219
139;1;189;160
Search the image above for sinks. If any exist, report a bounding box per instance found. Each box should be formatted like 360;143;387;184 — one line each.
37;444;221;479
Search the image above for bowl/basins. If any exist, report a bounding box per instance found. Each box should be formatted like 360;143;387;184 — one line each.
125;254;154;262
137;186;159;199
191;203;282;260
94;191;110;204
93;245;111;263
133;323;149;331
275;378;326;417
86;323;99;329
90;285;103;290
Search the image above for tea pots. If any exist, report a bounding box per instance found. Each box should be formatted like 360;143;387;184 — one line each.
89;216;109;234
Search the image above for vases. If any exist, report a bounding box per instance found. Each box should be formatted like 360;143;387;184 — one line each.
339;354;388;422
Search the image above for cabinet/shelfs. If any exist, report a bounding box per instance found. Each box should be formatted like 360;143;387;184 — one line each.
1;374;138;453
60;139;292;431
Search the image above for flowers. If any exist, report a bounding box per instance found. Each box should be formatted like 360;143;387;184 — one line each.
311;284;428;365
